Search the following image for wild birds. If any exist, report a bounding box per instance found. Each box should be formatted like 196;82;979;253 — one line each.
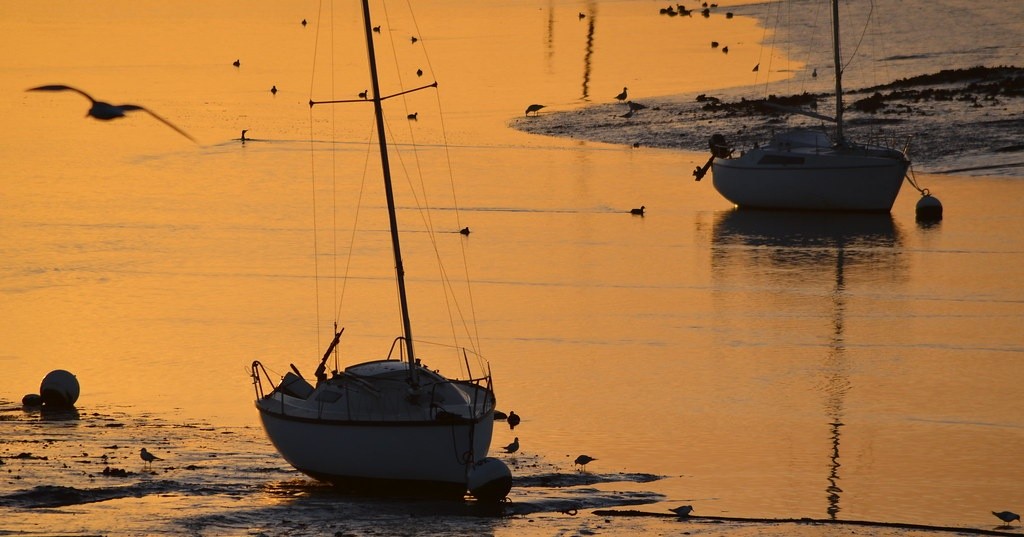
271;86;277;92
992;510;1021;526
25;84;197;144
578;12;586;17
711;40;729;54
657;0;736;20
525;104;547;117
501;437;520;458
233;59;240;66
460;226;470;234
812;68;818;77
301;19;307;24
240;129;249;140
668;505;694;517
613;86;648;121
139;447;160;468
574;454;599;473
507;411;520;430
360;25;423;120
630;206;646;214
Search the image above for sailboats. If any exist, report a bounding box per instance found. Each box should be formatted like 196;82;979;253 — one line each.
248;2;497;487
693;0;912;214
